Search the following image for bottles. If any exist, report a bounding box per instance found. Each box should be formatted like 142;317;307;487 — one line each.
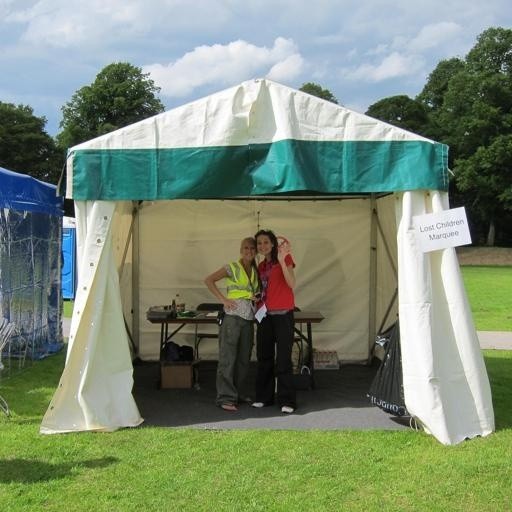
171;293;182;319
314;351;340;364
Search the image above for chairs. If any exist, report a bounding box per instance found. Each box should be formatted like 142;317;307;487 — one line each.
195;303;224;358
293;307;302;369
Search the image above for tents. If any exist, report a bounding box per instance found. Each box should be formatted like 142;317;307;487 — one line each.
37;77;496;446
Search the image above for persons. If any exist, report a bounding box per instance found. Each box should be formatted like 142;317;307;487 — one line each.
203;229;296;416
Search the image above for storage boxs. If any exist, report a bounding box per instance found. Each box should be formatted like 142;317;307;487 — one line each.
161;362;194;389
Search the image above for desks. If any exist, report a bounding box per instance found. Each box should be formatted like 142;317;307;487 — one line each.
148;311;325;382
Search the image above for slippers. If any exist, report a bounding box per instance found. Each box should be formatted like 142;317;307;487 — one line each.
221;404;237;411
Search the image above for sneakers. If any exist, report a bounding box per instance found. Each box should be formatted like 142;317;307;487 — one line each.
281;405;295;413
252;402;265;408
